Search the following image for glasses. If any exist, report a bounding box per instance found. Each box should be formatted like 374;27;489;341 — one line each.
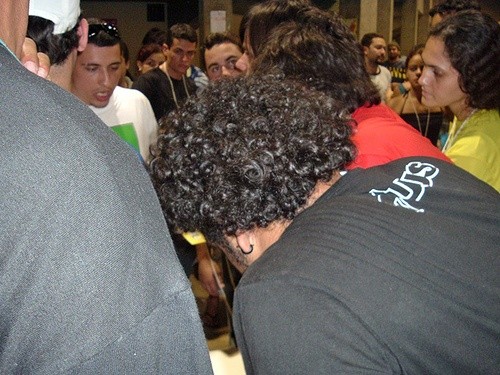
87;24;119;38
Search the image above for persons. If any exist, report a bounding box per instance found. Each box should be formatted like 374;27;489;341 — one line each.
0;1;213;375
71;18;159;163
145;63;500;375
28;0;500;357
250;7;455;170
418;10;500;194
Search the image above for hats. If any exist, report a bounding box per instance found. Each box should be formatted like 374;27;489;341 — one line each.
29;1;81;34
